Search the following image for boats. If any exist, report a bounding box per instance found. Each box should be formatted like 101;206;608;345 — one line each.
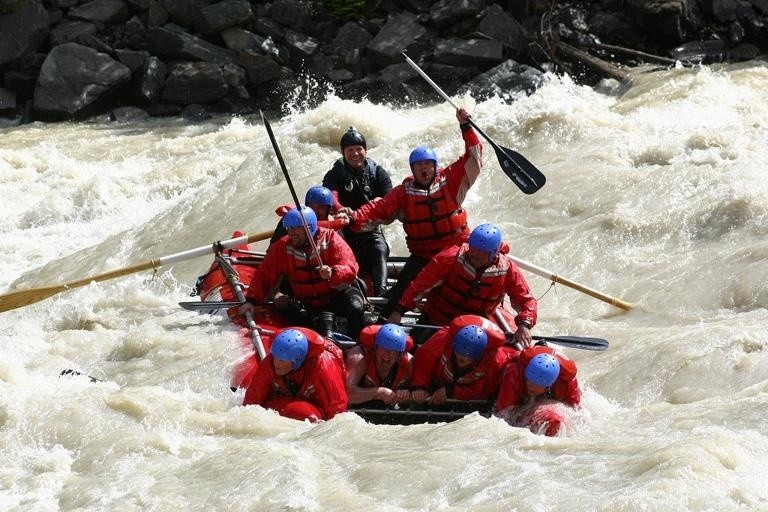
198;230;567;438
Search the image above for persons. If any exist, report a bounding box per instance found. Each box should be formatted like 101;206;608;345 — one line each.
242;326;347;420
333;107;483;324
493;346;579;436
266;185;345;240
322;130;393;298
386;224;537;354
236;204;366;346
344;325;417;404
410;314;506;406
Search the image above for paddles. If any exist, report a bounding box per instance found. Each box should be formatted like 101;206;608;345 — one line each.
178;296;427;311
0;230;276;313
398;322;609;351
258;110;323;267
396;48;546;194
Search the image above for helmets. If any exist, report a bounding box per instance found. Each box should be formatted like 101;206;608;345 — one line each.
451;325;488;358
375;324;406;361
469;224;501;264
340;130;366;155
525;352;560;388
283;206;318;237
270;330;308;372
305;187;333;218
410;148;437;175
